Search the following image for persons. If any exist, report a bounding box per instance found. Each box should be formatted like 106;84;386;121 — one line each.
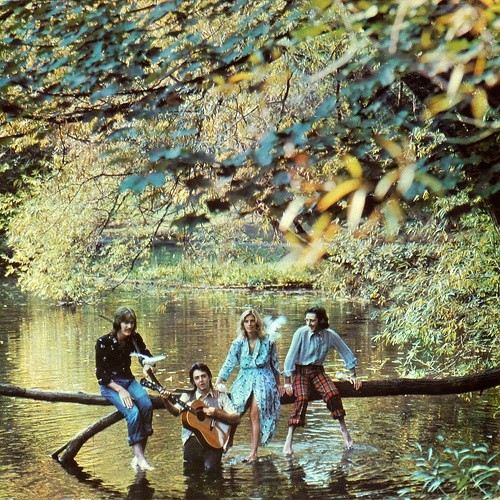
280;305;363;455
158;362;240;477
94;305;158;473
212;309;287;463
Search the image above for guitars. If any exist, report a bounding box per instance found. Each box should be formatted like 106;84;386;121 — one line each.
140;377;232;452
